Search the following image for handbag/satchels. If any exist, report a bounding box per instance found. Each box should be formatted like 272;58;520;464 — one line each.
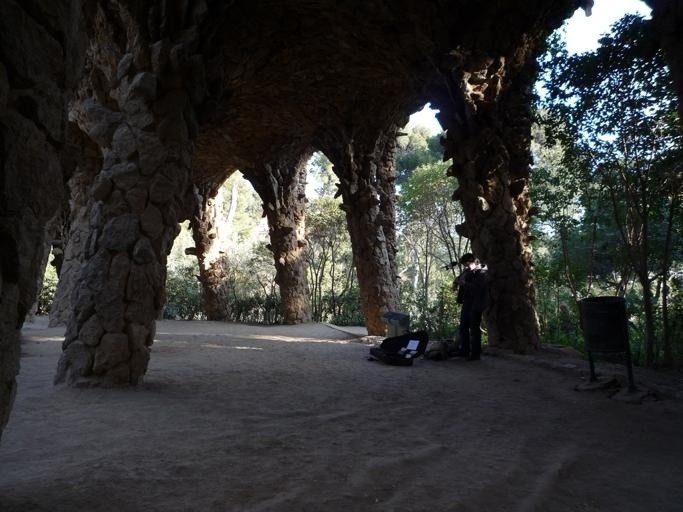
425;339;456;361
370;330;428;366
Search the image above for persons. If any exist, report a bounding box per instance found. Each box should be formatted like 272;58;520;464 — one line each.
451;254;487;362
